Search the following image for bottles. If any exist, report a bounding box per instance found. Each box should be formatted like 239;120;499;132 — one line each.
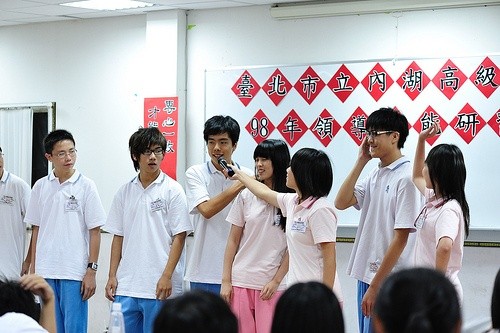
109;303;125;333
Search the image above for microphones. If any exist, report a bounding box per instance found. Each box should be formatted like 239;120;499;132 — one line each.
218;156;234;177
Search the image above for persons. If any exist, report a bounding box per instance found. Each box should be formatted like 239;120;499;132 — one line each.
410;118;471;315
23;129;105;333
0;273;57;333
151;265;500;333
0;146;31;285
183;115;255;301
102;126;195;333
334;107;424;333
222;147;345;318
220;138;291;333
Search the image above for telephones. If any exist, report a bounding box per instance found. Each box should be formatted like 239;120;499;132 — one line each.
203;52;500;248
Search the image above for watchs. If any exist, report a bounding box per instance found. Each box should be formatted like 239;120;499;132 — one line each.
87;261;98;271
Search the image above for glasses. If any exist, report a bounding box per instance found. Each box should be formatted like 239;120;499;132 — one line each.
142;147;165;154
52;149;77;158
0;153;4;156
366;130;400;137
